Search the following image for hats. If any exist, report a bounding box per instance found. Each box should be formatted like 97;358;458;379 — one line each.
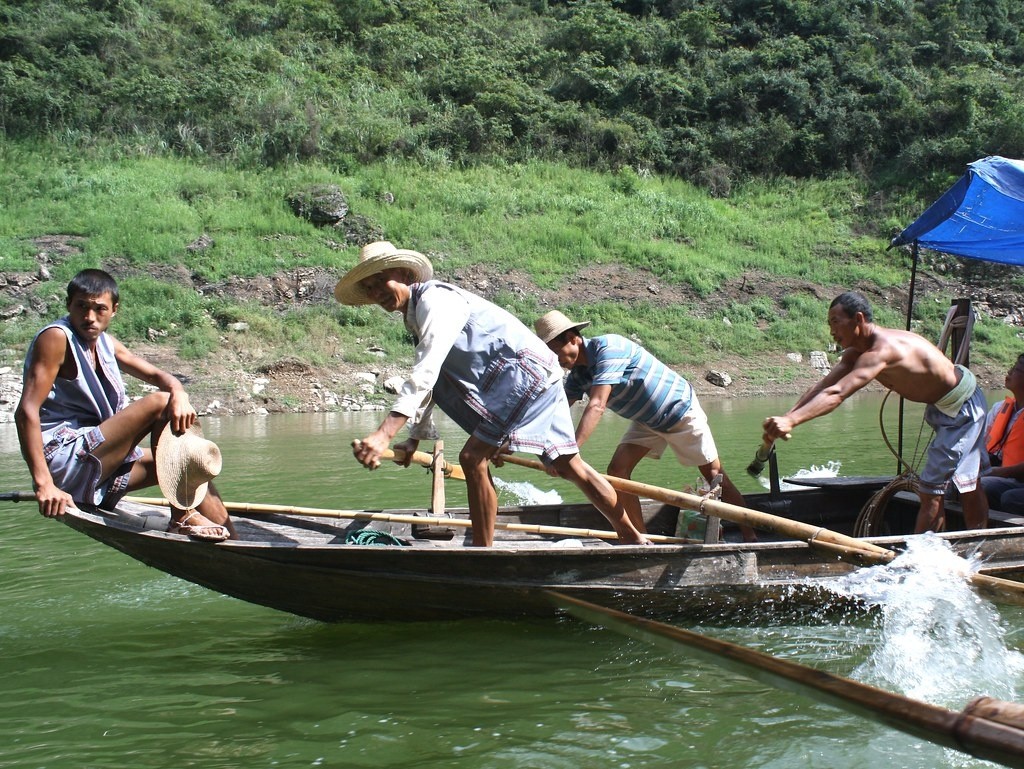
535;310;589;344
334;241;434;305
155;420;222;510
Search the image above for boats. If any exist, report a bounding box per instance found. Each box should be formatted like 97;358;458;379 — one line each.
41;153;1023;625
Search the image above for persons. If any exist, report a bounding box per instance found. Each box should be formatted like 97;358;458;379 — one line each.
1000;488;1024;516
534;310;760;546
763;291;988;545
14;269;228;541
981;353;1024;509
333;239;652;547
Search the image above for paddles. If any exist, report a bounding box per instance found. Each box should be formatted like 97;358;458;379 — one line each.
350;438;546;500
745;432;775;477
540;589;1023;769
499;453;1024;607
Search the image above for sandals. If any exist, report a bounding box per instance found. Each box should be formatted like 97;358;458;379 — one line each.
167;511;227;540
